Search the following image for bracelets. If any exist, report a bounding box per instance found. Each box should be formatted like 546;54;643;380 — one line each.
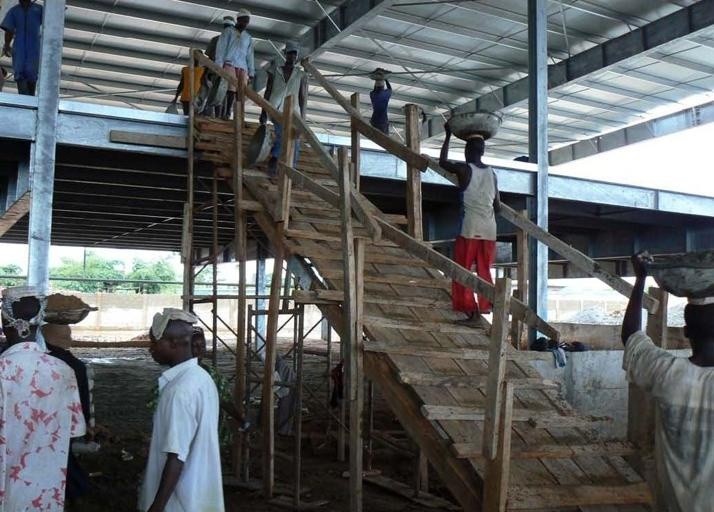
237;421;249;432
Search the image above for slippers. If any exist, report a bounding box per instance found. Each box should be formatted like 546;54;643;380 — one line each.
454;319;482;328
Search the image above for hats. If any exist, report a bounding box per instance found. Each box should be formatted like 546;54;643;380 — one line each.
375;80;384;87
237;9;250;19
286;41;298;54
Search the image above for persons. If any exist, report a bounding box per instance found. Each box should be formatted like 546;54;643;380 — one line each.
623;251;713;511
135;306;226;511
170;7;311;185
368;78;392;137
39;321;92;500
436;131;504;323
189;325;250;435
0;286;87;510
1;0;66;95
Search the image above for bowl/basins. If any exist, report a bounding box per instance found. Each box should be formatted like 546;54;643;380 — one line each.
207;74;228;107
634;246;713;297
366;72;392;80
162;104;178;115
246;125;273;168
42;307;99;325
444;108;503;139
